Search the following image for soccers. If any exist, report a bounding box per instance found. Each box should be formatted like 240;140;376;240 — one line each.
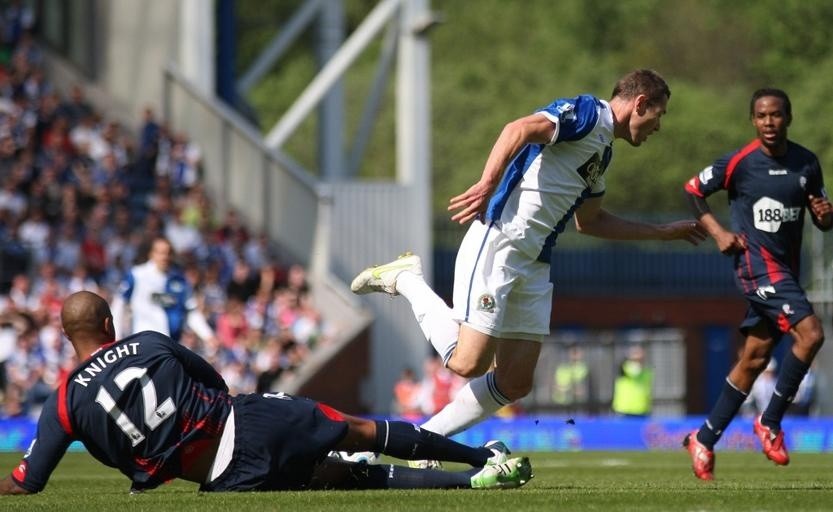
338;451;380;465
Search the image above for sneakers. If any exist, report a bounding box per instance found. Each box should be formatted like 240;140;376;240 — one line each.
407;460;445;472
328;448;378;465
350;251;423;300
483;438;509;467
683;429;715;481
752;412;790;466
478;455;535;488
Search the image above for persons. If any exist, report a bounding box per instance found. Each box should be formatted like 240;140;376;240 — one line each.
383;334;657;430
347;66;710;471
0;291;535;497
681;87;831;481
731;342;820;423
0;1;326;412
